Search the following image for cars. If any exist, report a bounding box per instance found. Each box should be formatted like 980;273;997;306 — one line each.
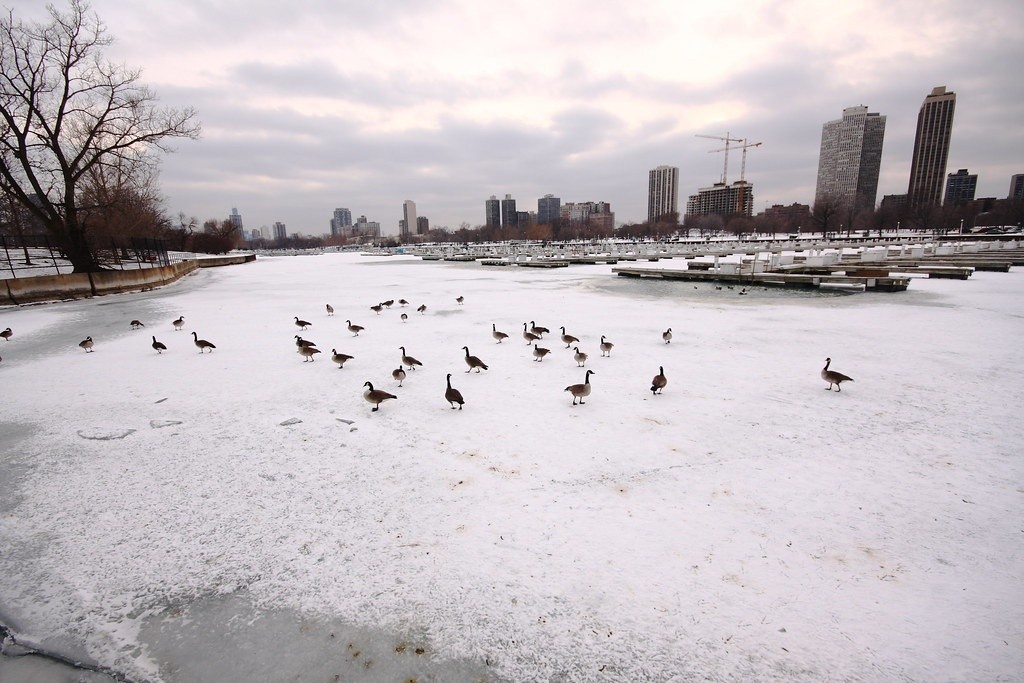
972;226;1018;234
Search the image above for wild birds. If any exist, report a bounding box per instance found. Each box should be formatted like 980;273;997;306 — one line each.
533;344;550;362
600;335;614;358
572;347;588;367
462;345;487;373
172;316;185;331
564;370;595;405
662;327;673;345
0;328;13;341
131;320;144;329
362;382;396;412
650;366;667;395
79;336;94;353
152;335;167;354
292;296;426;387
493;321;549;346
444;373;465;411
821;357;854;392
456;296;464;305
559;326;579;349
190;332;215;354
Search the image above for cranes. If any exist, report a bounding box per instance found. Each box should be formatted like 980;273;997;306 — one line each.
708;139;762;180
695;130;742;185
